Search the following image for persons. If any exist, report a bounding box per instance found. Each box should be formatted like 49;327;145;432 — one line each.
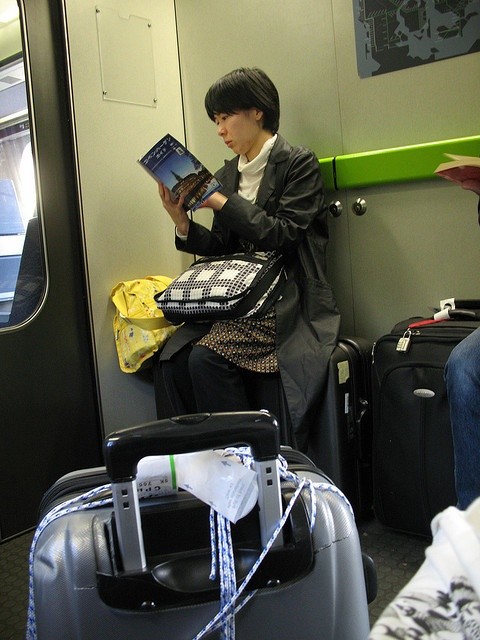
157;67;341;433
443;179;480;511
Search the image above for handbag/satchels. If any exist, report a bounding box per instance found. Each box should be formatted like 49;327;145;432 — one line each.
110;276;184;374
154;243;287;324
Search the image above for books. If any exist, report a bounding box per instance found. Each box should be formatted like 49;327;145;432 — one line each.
434;152;480;195
136;133;224;212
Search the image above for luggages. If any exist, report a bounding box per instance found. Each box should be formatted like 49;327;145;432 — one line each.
27;411;378;640
242;335;375;529
370;298;480;543
